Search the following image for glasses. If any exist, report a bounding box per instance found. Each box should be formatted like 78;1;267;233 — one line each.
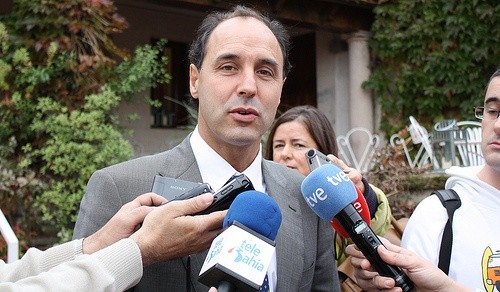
473;106;500;121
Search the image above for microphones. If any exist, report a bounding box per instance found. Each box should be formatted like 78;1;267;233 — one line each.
193;172;256;216
332;185;370;243
305;149;345;171
300;164;415;291
198;191;282;292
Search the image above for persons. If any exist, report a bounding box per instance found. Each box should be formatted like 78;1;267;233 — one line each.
400;68;500;292
338;218;485;292
264;105;391;265
71;6;341;292
0;191;230;292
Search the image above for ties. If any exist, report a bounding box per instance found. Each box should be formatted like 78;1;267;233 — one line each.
227;175;270;292
